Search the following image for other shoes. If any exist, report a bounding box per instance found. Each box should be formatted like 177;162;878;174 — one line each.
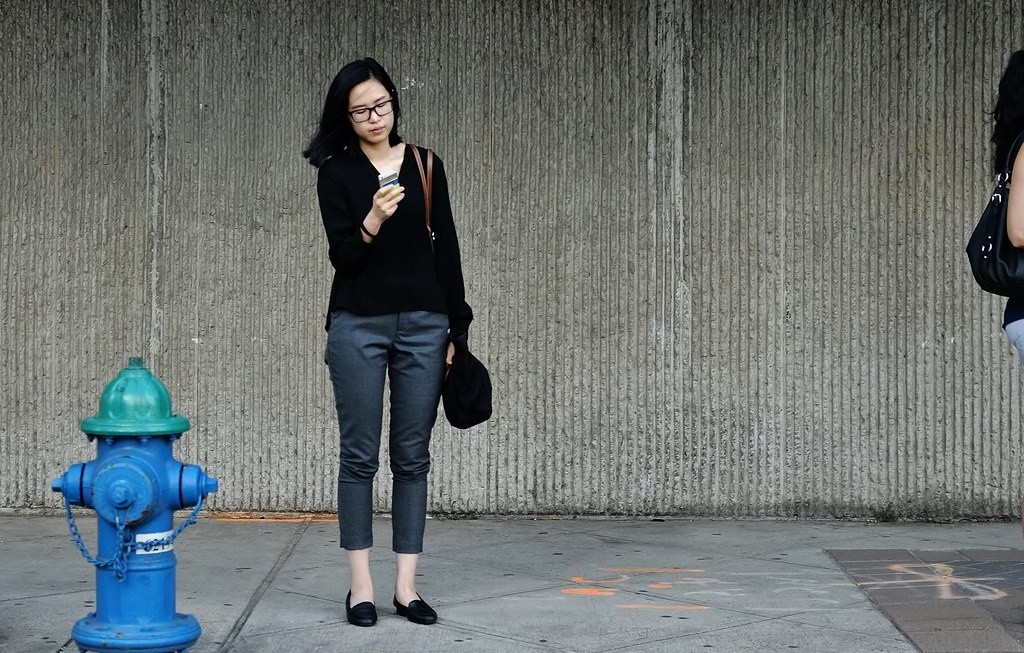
345;589;378;629
394;591;439;626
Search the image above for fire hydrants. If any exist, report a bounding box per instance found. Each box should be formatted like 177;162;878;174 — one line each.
51;354;220;653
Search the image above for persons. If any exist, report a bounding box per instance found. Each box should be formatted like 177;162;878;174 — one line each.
302;58;465;627
991;50;1024;362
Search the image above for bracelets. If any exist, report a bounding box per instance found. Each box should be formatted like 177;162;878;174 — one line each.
360;223;376;238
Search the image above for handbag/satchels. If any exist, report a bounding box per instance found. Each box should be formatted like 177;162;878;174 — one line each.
965;173;1023;299
440;344;494;430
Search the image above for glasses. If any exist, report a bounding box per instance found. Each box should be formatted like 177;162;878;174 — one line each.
347;98;397;123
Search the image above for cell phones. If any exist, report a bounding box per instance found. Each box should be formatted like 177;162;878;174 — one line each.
378;169;401;202
